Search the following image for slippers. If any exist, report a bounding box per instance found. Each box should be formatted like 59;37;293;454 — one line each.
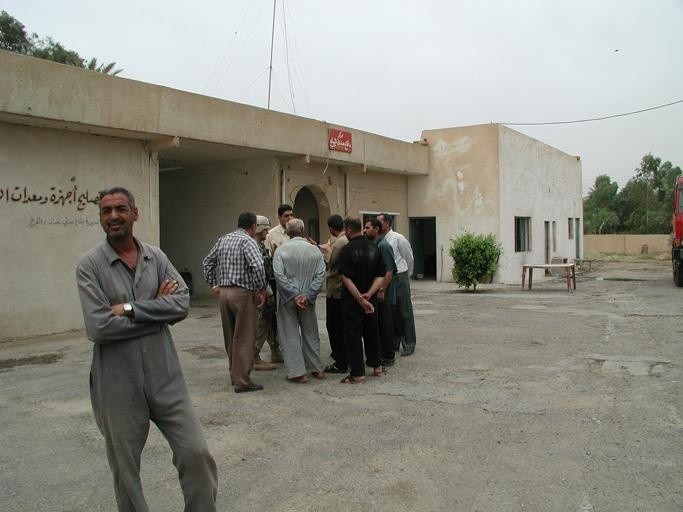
341;376;363;384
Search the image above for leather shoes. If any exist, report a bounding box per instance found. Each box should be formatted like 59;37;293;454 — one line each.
235;383;263;393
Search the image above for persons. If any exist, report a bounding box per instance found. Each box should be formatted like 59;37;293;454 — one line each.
336;216;386;383
204;212;267;391
265;206;294;262
377;213;415;356
76;188;218;512
251;215;284;370
363;218;397;367
273;218;326;383
318;215;349;374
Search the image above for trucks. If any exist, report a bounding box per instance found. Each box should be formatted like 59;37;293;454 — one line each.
669;173;683;287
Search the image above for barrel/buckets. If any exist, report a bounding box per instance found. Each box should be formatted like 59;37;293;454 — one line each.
551;258;568;277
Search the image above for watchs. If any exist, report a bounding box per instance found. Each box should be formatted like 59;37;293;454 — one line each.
123;303;132;316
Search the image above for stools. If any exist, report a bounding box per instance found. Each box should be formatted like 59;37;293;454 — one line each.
573;258;592;272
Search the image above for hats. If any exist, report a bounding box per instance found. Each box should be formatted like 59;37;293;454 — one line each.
255;215;270;233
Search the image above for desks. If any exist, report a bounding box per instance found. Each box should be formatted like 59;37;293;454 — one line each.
520;263;576;294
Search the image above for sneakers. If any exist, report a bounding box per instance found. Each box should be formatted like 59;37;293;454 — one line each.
324;365;348;373
